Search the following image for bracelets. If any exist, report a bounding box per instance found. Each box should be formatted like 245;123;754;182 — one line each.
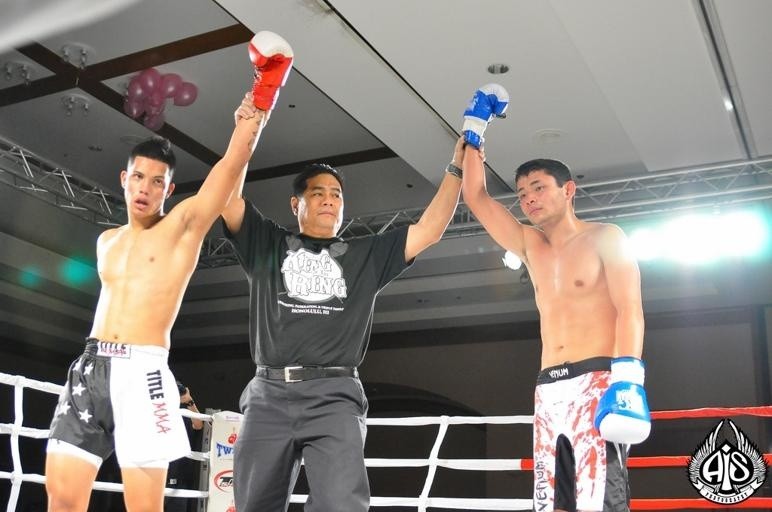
446;163;462;179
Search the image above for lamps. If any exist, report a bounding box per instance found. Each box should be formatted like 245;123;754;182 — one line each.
59;40;96;68
61;95;98;120
1;58;37;86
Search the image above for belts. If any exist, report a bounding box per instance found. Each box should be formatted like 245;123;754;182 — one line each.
256;366;358;383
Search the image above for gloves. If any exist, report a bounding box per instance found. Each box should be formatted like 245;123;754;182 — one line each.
248;31;294;111
462;83;509;149
594;356;652;444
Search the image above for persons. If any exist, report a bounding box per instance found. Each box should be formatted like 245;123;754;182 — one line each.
461;83;652;511
45;31;293;512
165;379;203;512
222;92;485;511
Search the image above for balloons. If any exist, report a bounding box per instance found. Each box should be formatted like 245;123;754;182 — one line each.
123;68;197;132
